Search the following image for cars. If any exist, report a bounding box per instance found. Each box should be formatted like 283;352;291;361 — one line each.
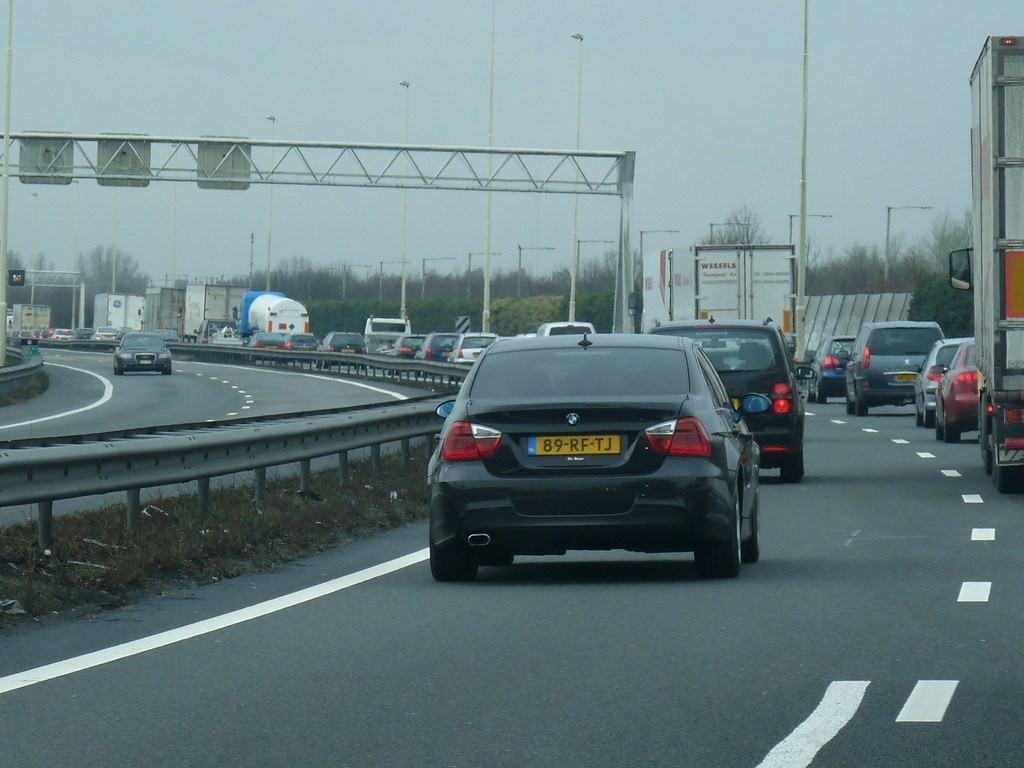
387;331;497;372
246;330;367;368
7;326;180;374
929;340;979;443
425;332;774;578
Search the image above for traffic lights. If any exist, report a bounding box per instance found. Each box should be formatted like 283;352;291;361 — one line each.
7;270;25;287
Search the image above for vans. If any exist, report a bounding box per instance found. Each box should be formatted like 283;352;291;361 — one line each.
364;314;411;357
194;319;237;347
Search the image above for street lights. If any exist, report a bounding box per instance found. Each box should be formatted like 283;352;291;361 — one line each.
268;32;585;330
305;239;614;306
640;230;681;257
788;215;831;243
710;223;750;241
886;205;935;283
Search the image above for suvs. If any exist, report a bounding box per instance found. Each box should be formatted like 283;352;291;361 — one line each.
536;321;594;339
644;313;818;481
807;319;975;428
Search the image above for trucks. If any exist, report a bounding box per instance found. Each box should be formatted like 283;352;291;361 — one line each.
949;36;1024;492
628;243;797;360
6;283;310;347
